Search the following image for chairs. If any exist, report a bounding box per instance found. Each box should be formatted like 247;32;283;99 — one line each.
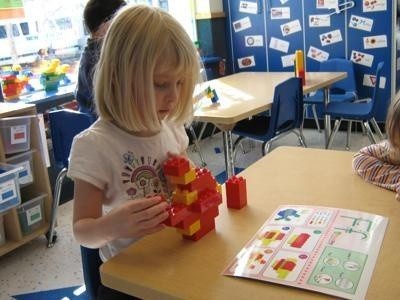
193;56;233;153
79;244;104;300
47;109;97;248
325;63;384;149
229;78;306;162
301;58;366;137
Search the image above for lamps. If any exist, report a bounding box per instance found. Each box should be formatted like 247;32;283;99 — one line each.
1;0;24;56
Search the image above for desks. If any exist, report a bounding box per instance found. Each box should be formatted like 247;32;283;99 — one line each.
193;72;347;179
98;145;400;300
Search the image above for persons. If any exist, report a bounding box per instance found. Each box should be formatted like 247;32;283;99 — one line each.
75;1;129;115
352;90;400;199
71;5;201;297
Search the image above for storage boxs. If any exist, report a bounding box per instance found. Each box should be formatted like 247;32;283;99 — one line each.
5;148;38;189
0;163;23;214
0;115;37;155
15;189;48;237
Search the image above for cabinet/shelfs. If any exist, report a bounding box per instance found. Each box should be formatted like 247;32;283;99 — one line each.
222;0;400;134
0;102;57;257
5;84;76;150
